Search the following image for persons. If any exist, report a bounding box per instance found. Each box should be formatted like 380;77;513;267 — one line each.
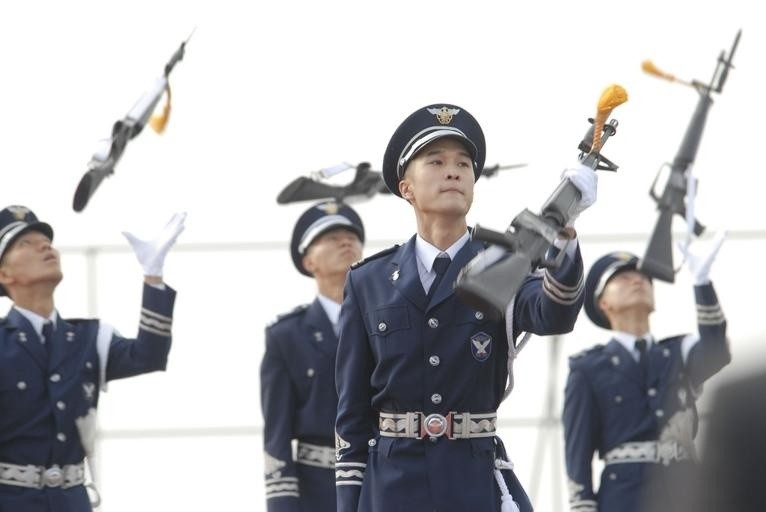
0;204;187;512
260;200;364;511
332;104;598;512
560;227;766;511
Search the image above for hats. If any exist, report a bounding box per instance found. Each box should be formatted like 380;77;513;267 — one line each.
0;206;53;259
382;105;486;199
291;201;364;278
584;252;653;329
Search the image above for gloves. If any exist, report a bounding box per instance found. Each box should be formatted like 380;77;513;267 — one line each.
122;212;187;276
562;164;597;228
676;229;728;286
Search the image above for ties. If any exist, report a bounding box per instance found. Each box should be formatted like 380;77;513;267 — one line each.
635;340;646;364
426;258;450;299
43;322;54;351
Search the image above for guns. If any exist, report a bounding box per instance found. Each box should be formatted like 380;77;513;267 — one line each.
451;118;618;325
635;28;742;283
73;26;198;211
277;162;527;204
